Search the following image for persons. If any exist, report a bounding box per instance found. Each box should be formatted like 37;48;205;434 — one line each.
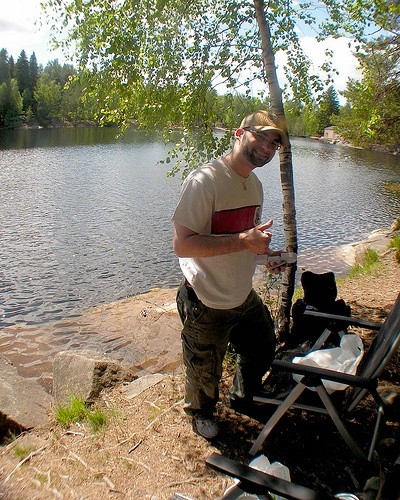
169;111;289;439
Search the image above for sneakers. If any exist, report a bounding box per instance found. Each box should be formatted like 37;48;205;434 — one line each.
230;392;272;409
194;413;220;438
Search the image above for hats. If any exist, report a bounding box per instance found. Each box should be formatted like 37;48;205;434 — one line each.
239;110;290;147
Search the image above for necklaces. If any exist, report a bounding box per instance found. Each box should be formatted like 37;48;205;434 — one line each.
225;158;250;191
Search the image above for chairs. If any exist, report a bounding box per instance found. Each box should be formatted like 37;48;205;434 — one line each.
168;451;400;500
245;290;400;470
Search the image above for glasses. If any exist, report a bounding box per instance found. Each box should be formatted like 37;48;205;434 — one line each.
245;129;282;150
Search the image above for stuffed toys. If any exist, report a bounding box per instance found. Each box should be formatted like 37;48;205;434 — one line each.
278;271;350;346
